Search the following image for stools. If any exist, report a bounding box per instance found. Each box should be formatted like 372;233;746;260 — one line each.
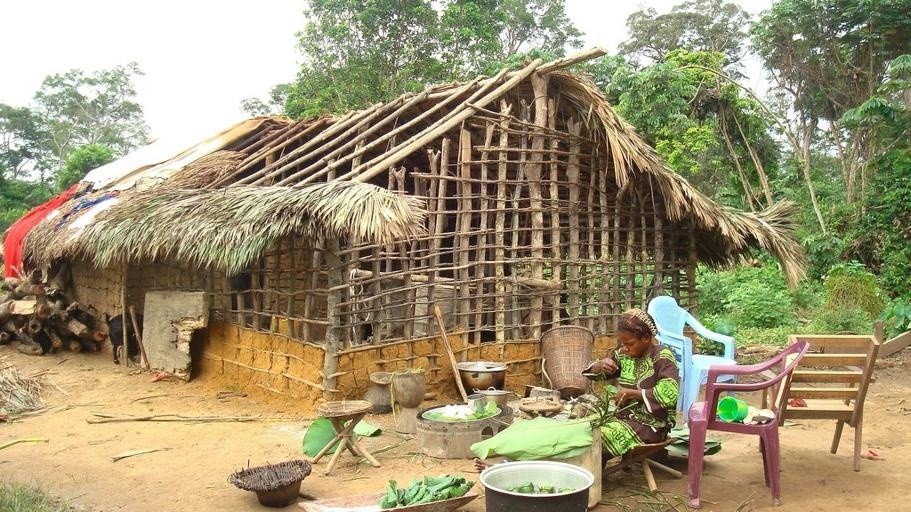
312;400;381;475
605;436;682;492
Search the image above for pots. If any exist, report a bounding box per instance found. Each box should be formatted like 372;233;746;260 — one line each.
473;388;513;409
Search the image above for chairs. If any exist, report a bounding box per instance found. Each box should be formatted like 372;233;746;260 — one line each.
758;334;880;472
687;341;809;508
648;295;738;423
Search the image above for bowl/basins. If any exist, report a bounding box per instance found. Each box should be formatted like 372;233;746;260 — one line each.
455;362;508;390
479;461;594;512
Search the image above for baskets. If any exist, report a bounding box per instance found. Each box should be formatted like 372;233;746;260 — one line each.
317;399;374;420
520;399;562;413
540;325;595;398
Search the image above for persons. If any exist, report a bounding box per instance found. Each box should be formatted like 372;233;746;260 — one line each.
471;307;680;476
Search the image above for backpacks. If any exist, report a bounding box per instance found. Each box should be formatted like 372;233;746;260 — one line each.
228;458;311;507
